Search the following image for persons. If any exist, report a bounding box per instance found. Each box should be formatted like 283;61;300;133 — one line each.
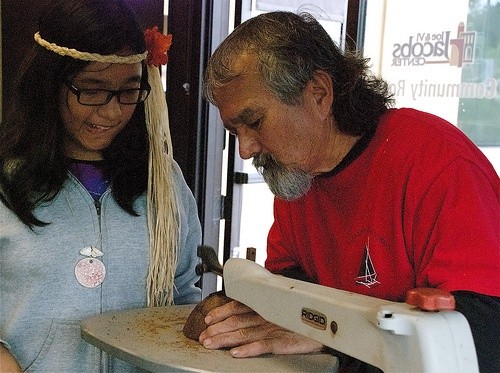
199;10;499;373
0;0;205;373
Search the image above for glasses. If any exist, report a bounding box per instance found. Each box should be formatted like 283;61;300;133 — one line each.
64;79;151;106
